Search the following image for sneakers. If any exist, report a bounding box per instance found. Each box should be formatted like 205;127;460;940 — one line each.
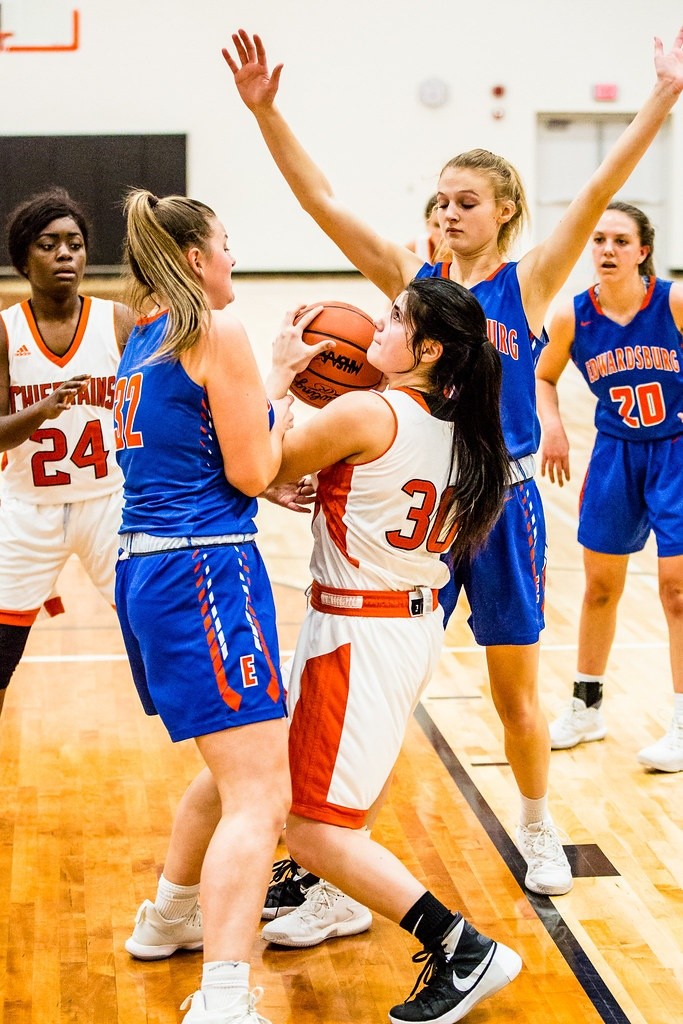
388;911;523;1024
180;986;273;1024
126;898;202;960
637;720;683;772
262;855;320;919
261;878;374;947
516;818;573;895
545;697;606;749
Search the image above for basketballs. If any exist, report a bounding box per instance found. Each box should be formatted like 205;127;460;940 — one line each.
288;300;385;410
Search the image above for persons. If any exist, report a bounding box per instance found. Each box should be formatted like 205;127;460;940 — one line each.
263;279;524;1024
0;187;319;714
406;196;444;266
223;27;682;948
535;203;682;775
112;189;293;1023
